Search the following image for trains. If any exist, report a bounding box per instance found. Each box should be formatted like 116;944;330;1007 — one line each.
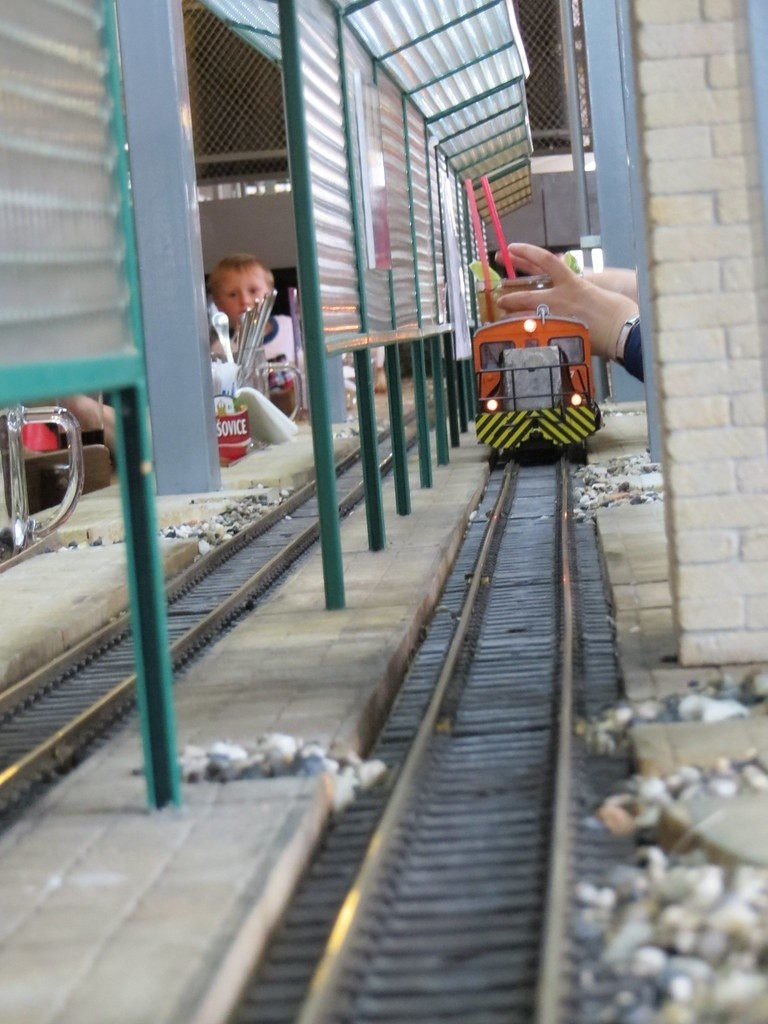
472;304;604;467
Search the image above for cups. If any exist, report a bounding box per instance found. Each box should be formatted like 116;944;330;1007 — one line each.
209;346;303;426
475;273;581;332
0;402;83;564
209;360;252;470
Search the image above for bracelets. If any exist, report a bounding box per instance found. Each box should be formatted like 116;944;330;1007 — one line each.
615;314;639;362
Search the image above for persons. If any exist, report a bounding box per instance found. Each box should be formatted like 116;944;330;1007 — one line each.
209;253;308;419
22;394;115;458
495;242;645;383
